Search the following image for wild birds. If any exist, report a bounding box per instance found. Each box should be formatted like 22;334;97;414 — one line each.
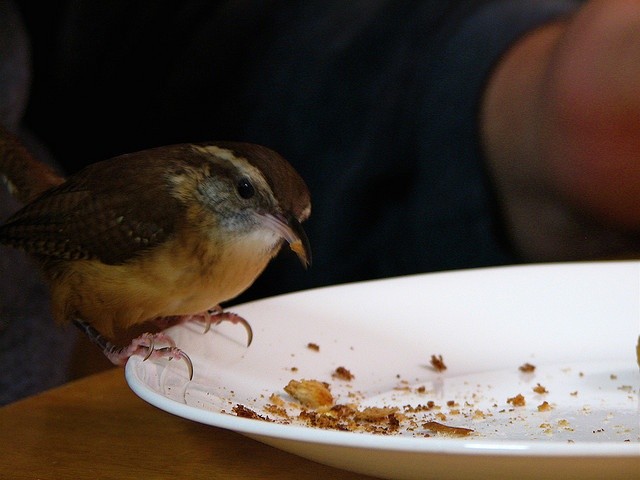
1;126;319;383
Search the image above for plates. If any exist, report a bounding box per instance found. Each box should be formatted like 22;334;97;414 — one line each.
122;259;640;480
